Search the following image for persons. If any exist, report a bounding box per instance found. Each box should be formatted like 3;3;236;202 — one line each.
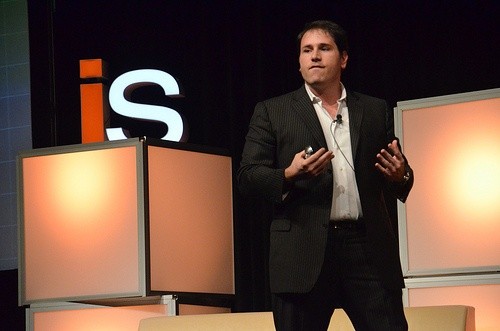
236;18;414;331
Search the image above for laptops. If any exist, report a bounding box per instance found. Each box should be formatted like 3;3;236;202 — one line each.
106;127;129;141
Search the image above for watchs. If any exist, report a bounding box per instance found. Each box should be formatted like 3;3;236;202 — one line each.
398;171;411;186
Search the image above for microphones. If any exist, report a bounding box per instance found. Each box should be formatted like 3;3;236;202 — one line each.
333;114;343;124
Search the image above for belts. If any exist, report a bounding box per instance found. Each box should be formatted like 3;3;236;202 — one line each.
328;220;363;235
